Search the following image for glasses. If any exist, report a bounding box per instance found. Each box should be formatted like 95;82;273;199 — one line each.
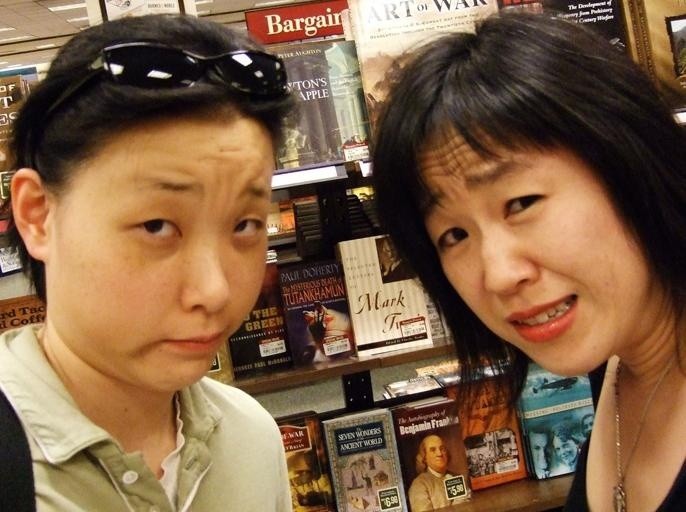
46;42;286;109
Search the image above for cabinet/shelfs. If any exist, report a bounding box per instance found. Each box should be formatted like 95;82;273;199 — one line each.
227;334;575;512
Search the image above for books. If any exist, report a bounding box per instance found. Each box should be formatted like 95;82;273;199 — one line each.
1;67;46;334
206;1;686;382
265;354;599;510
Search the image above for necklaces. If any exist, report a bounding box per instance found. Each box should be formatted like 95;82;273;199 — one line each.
608;359;675;512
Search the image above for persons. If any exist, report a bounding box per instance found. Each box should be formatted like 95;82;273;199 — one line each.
0;13;292;512
360;9;686;512
